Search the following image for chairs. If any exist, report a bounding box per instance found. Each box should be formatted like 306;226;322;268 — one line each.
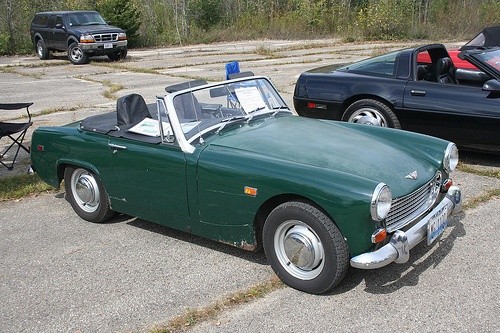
0;102;33;171
172;91;204;120
226;61;271;111
117;93;161;142
434;57;458;87
414;66;429;84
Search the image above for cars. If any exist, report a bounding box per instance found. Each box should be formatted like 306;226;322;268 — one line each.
414;26;500;71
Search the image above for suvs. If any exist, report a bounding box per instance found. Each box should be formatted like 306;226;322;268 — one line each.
29;10;128;65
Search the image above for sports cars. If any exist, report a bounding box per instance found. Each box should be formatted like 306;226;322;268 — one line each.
291;26;500;158
28;70;463;295
